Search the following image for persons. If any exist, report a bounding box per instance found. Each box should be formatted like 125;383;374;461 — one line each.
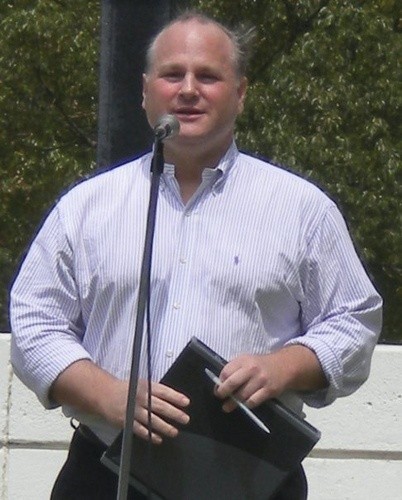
6;11;384;500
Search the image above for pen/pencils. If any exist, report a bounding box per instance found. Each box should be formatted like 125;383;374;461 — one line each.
205;367;272;434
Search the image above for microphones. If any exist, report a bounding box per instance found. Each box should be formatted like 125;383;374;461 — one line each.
153;113;181;140
